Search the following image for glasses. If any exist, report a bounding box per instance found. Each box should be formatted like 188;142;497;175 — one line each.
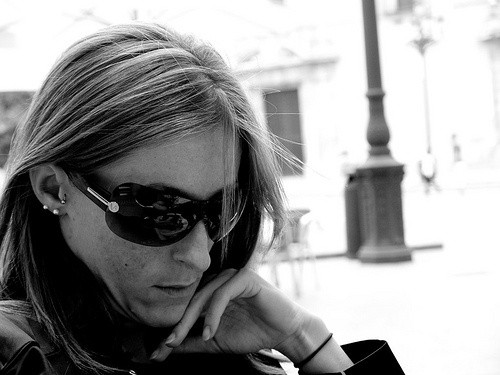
72;176;251;248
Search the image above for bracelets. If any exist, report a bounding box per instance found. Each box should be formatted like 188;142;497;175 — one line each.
294;326;333;369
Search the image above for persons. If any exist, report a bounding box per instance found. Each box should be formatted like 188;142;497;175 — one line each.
1;22;407;375
